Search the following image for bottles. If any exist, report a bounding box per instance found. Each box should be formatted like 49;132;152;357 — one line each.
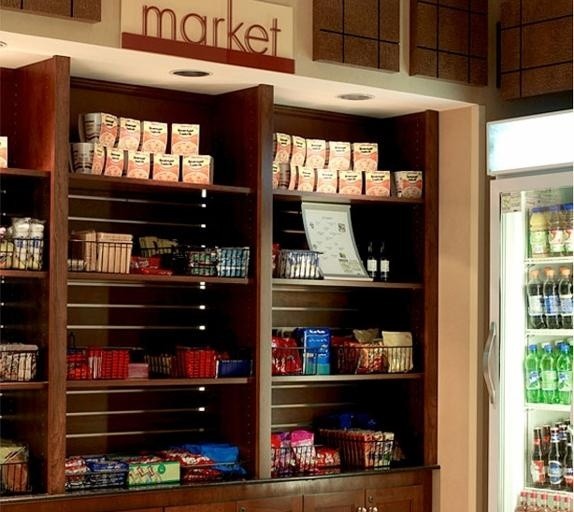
525;197;573;494
363;236;394;281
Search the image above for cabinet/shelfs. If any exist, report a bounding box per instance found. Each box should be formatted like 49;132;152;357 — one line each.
0;464;432;512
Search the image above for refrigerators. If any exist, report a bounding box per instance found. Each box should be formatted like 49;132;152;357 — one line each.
483;107;573;511
